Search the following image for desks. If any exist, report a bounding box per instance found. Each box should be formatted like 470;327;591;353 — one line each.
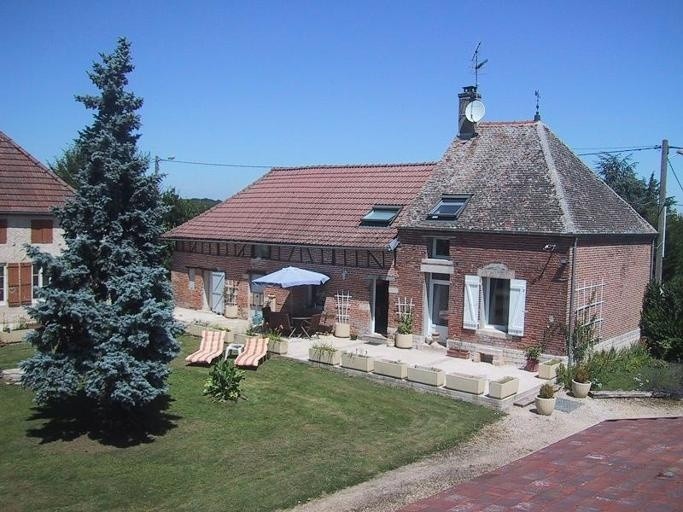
224;341;244;363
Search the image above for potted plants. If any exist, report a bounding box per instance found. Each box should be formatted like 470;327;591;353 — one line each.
570;367;591;397
392;312;415;349
525;346;539;373
534;384;555;416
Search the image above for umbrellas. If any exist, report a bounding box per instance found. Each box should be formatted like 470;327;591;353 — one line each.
250;265;329;328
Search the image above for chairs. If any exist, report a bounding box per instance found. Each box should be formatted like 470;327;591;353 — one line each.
234;337;270;369
259;303;322;338
183;329;226;366
